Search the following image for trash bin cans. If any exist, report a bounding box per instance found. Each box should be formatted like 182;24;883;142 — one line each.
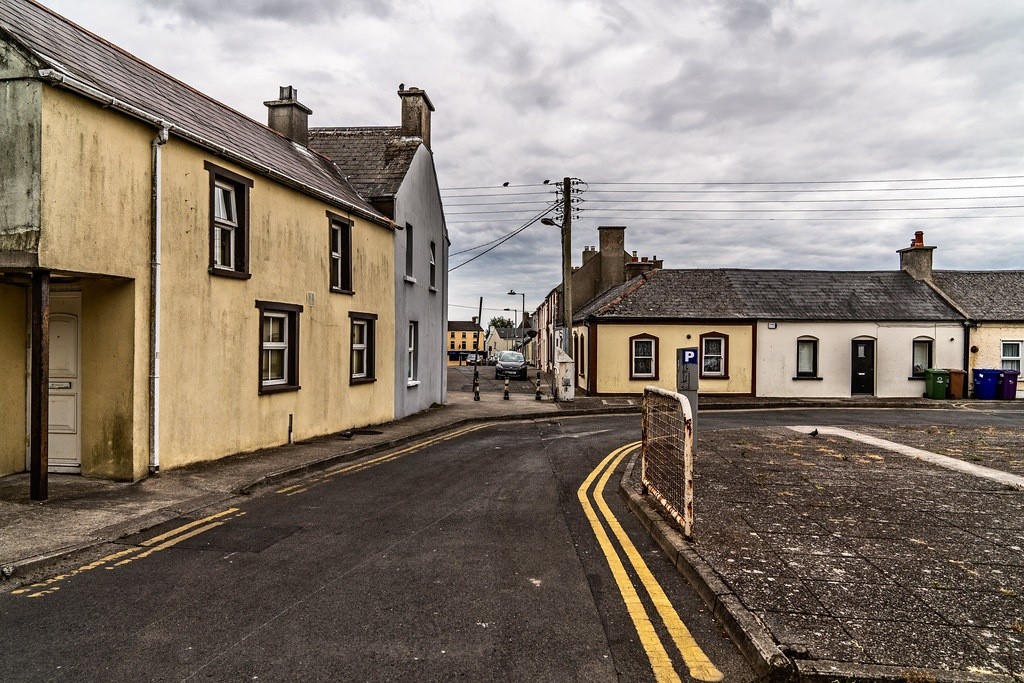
943;368;967;400
994;369;1021;400
923;368;949;400
970;368;1002;400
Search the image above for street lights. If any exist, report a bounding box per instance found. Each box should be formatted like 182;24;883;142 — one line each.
506;289;525;355
504;308;517;351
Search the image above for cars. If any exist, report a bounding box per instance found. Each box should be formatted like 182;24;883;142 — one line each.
488;350;530;380
465;353;483;367
